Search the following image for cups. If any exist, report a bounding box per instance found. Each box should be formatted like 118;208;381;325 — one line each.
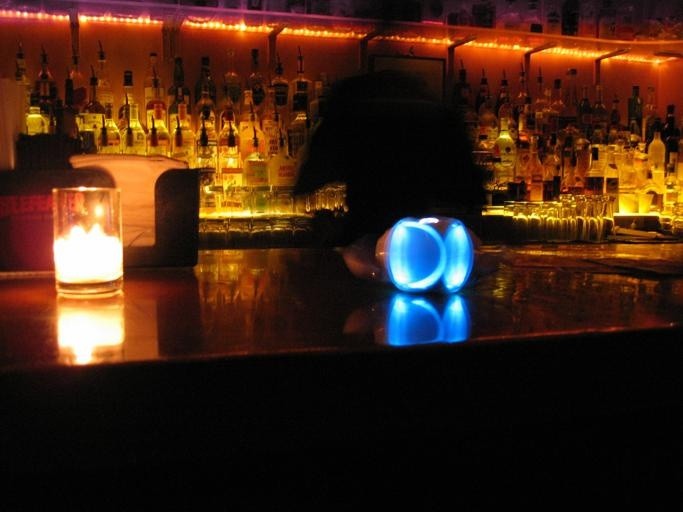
53;288;126;363
50;186;126;292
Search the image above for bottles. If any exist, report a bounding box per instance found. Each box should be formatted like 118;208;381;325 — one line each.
13;46;682;218
